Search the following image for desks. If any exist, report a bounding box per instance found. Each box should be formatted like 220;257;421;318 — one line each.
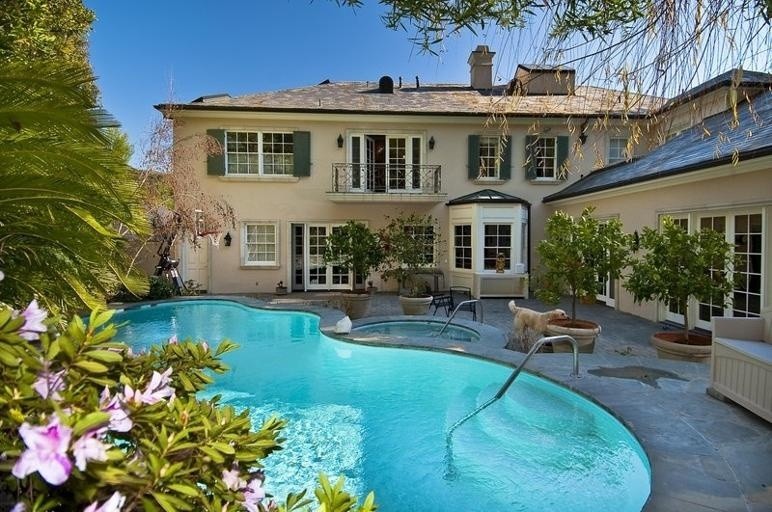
397;268;441;296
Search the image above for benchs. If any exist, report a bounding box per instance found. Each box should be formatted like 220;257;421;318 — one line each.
710;316;772;424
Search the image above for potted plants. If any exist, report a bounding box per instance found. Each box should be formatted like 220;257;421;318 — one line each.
323;220;386;320
374;208;446;315
520;204;629;354
621;215;751;363
367;281;377;294
276;280;287;294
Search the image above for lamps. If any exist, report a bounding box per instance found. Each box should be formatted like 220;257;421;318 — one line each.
224;232;231;246
337;134;343;147
429;136;434;149
579;129;586;144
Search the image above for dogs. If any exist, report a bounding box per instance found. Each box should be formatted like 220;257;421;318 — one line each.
507;299;570;350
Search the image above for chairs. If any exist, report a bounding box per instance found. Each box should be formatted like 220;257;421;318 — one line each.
447;285;476;321
425;282;453;316
